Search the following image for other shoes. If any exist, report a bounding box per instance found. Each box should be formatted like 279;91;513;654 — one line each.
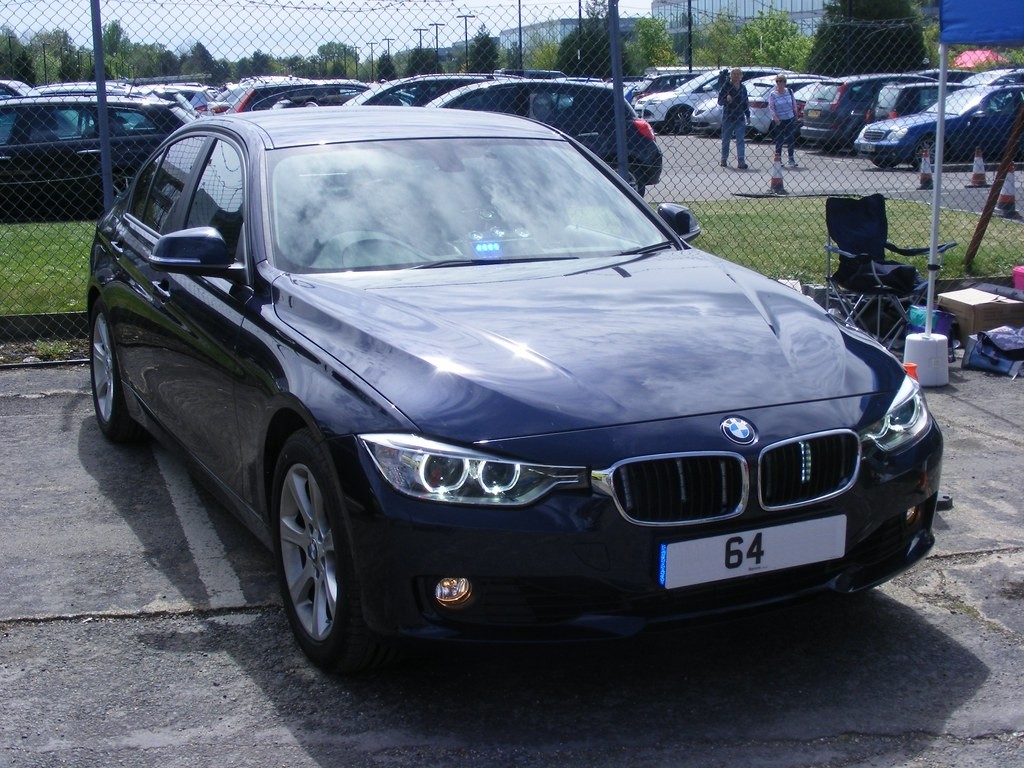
738;163;748;169
788;159;798;167
773;162;784;168
721;160;727;167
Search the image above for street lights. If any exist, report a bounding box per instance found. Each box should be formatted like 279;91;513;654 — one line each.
90;52;137;78
366;43;378;83
42;42;51;85
413;28;427;74
429;23;445;74
382;39;395;80
275;45;361;79
457;15;476;74
8;36;16;76
60;47;68;83
751;27;763;53
77;51;83;82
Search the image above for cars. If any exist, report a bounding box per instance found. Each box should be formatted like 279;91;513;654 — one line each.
84;106;944;678
0;97;197;212
865;82;972;124
424;81;663;196
854;84;1024;172
0;77;303;138
632;65;831;140
340;74;555;120
958;69;1024;85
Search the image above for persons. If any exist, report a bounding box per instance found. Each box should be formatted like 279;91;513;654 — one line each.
717;68;751;170
769;73;798;167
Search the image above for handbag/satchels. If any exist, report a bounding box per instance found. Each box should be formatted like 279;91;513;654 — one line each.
794;121;804;139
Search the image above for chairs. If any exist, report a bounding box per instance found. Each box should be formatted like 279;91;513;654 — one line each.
825;193;958;354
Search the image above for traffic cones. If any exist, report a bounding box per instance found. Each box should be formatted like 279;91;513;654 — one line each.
992;161;1020;218
767;153;790;196
964;146;992;187
915;149;934;190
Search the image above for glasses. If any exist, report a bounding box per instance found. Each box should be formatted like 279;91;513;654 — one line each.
776;80;784;83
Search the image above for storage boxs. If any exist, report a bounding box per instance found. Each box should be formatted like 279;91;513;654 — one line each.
908;306;954;352
936;288;1024;348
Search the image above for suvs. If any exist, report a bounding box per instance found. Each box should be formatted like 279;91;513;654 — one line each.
801;72;939;154
